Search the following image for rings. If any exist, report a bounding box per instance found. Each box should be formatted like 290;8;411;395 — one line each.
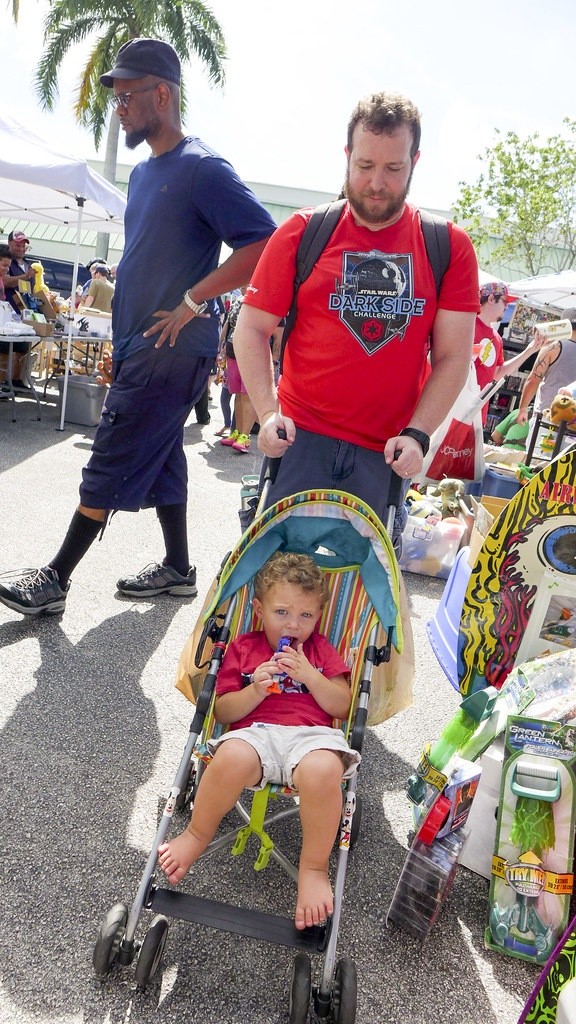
406;472;409;475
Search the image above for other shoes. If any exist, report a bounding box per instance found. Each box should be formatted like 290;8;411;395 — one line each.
198;416;211;425
214;426;232;436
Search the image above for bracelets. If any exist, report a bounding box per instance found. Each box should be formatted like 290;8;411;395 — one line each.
273;360;279;366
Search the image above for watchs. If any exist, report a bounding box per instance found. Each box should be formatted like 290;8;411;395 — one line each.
183;289;207;315
399;428;430;457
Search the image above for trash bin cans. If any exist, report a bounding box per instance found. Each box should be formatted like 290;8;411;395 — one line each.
55;374;108;429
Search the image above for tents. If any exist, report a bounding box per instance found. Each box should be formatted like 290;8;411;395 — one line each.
478;270;576;317
0;111;127;432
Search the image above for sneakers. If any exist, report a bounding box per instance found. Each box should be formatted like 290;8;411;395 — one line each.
232;433;252;453
115;560;198;598
2;378;35;393
0;385;13;398
0;564;73;615
220;429;241;446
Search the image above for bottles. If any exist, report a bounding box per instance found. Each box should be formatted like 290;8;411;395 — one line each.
241;475;260;510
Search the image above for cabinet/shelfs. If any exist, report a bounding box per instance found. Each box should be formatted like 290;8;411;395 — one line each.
485;340;539;441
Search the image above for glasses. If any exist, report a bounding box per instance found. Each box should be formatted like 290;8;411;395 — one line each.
494;296;508;306
109;86;158;110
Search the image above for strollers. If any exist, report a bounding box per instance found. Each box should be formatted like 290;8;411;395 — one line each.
89;427;406;1024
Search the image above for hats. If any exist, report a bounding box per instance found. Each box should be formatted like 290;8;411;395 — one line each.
478;282;519;303
99;38;181;89
8;231;30;245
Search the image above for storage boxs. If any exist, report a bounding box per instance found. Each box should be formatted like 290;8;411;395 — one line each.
13;290;59;335
56;373;109;426
396;467;521;695
74;308;113;338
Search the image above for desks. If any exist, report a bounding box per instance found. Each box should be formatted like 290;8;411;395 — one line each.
0;336;113;423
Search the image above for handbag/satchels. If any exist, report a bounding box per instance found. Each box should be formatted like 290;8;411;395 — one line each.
409;358;485;486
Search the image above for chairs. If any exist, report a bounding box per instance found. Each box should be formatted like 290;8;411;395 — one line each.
30;341;112;379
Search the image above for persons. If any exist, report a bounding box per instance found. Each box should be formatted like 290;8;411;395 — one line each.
0;37;280;614
491;404;533;452
427;280;547;486
516;307;576;458
194;282;287;453
233;92;479;560
158;554;361;929
0;231;119;400
